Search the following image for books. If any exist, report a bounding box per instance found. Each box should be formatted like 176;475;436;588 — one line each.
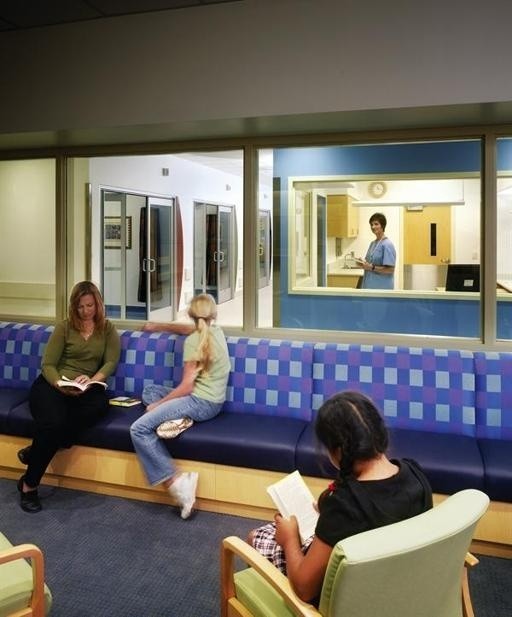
109;396;142;407
58;375;108;392
266;469;321;545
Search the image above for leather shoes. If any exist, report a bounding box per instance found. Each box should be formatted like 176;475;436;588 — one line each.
18;446;32;464
18;476;42;513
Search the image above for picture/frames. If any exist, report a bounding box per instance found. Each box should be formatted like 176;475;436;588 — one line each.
104;216;132;249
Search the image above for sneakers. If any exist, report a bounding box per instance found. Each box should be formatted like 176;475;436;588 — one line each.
167;471;198;521
156;416;194;440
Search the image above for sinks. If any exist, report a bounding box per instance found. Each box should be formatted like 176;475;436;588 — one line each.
342;266;363;270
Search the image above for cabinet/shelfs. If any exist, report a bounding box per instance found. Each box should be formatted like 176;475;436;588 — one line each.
326;193;359;238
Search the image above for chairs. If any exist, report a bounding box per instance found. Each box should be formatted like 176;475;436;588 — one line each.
0;532;54;617
220;488;491;617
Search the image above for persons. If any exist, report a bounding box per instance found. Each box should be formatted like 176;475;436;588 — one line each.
17;280;122;513
129;293;231;520
355;213;396;289
248;390;433;610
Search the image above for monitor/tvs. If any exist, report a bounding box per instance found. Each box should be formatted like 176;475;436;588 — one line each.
445;263;480;292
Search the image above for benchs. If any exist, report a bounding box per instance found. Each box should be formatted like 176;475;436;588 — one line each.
0;316;512;562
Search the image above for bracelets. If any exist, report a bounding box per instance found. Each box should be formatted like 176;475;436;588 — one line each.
372;263;375;271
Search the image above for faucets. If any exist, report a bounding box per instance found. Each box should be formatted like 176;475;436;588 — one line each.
343;253;352;264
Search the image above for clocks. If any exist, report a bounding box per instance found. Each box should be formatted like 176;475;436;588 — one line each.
368;182;387;197
